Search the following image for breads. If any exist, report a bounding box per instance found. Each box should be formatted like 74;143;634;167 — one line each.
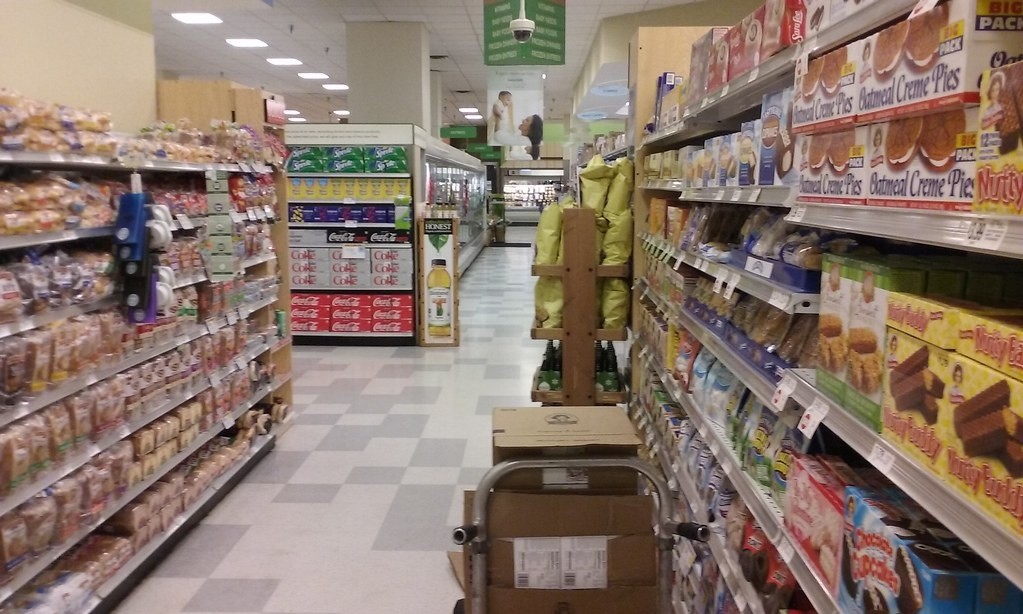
0;87;289;614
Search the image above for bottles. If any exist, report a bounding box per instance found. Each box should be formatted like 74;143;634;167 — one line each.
427;259;452;337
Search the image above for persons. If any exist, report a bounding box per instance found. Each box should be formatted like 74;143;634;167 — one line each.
887;334;899;373
949;365;965;405
860;42;872;82
981;72;1004;132
506;145;540;161
843;495;856;537
487;90;544;146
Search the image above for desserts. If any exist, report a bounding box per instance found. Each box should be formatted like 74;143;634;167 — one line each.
693;5;1022;614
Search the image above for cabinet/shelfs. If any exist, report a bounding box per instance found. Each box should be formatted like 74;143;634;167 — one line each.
159;80;284;142
0;149;293;614
633;0;1023;614
531;209;631;407
282;122;485;349
503;185;558;226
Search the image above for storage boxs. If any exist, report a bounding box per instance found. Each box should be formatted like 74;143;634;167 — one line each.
286;178;411;201
821;260;1023;539
651;198;692;239
290;205;413;224
448;407;662;614
287;248;415;289
289;229;328;244
643;0;1023;216
640;301;670;357
290;293;416;333
784;452;1023;614
641;363;688;449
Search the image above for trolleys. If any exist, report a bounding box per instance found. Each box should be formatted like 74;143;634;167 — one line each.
450;455;716;614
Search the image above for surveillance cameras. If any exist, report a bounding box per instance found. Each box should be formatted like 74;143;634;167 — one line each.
509;20;535;44
